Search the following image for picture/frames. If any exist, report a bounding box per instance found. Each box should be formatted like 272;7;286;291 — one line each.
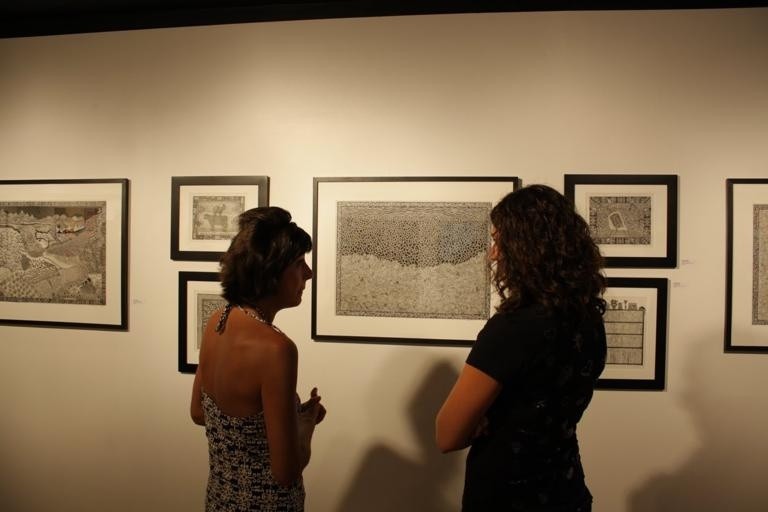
312;174;520;348
563;173;680;269
177;270;223;375
170;174;271;263
592;277;668;392
723;178;768;353
1;178;131;332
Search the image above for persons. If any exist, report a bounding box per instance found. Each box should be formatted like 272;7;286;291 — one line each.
435;184;607;512
190;207;326;512
